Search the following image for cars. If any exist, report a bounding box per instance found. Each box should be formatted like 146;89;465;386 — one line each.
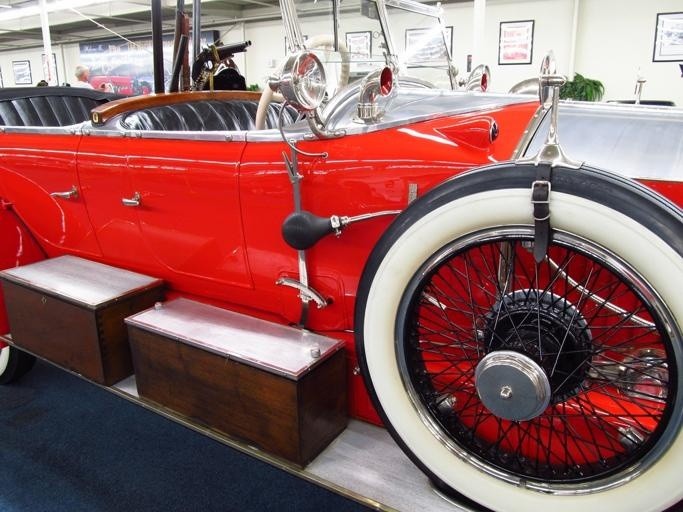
0;0;682;512
79;59;173;96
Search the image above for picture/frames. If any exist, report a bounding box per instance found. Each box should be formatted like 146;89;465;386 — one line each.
652;12;682;63
344;20;534;77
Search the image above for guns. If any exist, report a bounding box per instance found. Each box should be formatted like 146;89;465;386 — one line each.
192;41;252;81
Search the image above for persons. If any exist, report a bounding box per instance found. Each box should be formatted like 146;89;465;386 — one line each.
35;80;48;87
66;66;94;90
